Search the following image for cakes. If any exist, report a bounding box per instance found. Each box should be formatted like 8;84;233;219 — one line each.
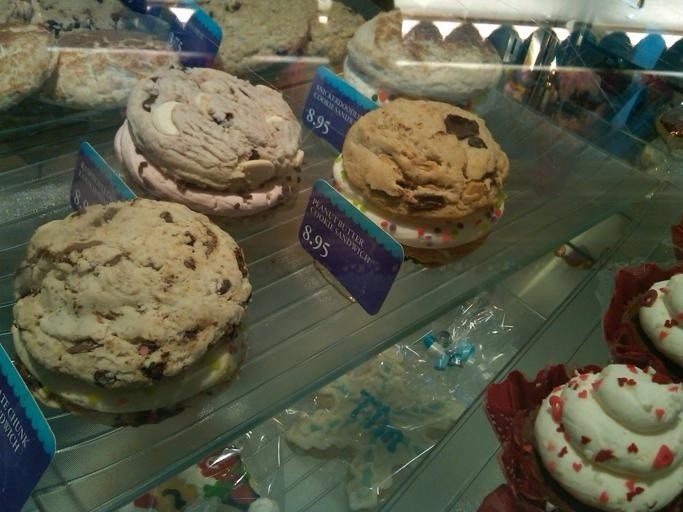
285;345;457;511
533;365;682;511
120;445;281;512
640;271;683;362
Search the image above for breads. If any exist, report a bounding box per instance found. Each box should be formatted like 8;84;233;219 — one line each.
334;101;508;267
200;0;309;74
114;68;302;214
34;30;178;111
298;0;367;64
1;22;60;108
12;198;253;428
343;10;504;116
0;1;128;34
